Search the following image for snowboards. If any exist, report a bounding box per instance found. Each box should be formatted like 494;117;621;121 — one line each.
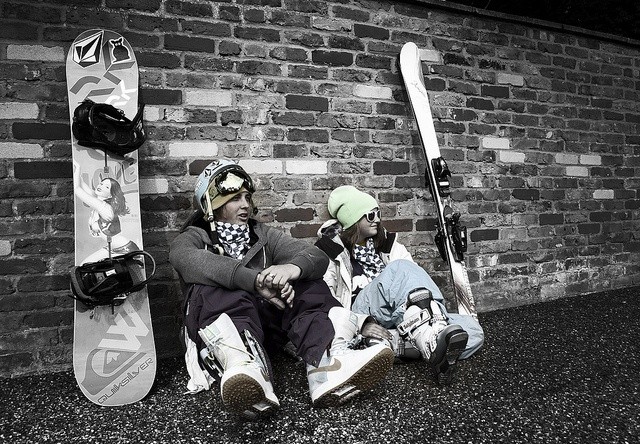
397;42;481;339
65;28;157;407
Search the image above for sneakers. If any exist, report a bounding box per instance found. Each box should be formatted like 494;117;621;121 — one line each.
353;326;419;359
403;292;466;384
305;308;393;407
198;315;279;417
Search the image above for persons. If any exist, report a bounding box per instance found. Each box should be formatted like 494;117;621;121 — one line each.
168;156;399;418
314;185;469;388
217;197;220;207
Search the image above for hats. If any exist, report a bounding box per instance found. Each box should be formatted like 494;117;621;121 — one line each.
328;185;378;231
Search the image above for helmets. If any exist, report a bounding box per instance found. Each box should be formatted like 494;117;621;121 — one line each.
194;159;255;208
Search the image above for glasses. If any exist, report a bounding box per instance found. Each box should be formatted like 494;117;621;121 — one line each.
204;168;255;203
363;210;382;222
